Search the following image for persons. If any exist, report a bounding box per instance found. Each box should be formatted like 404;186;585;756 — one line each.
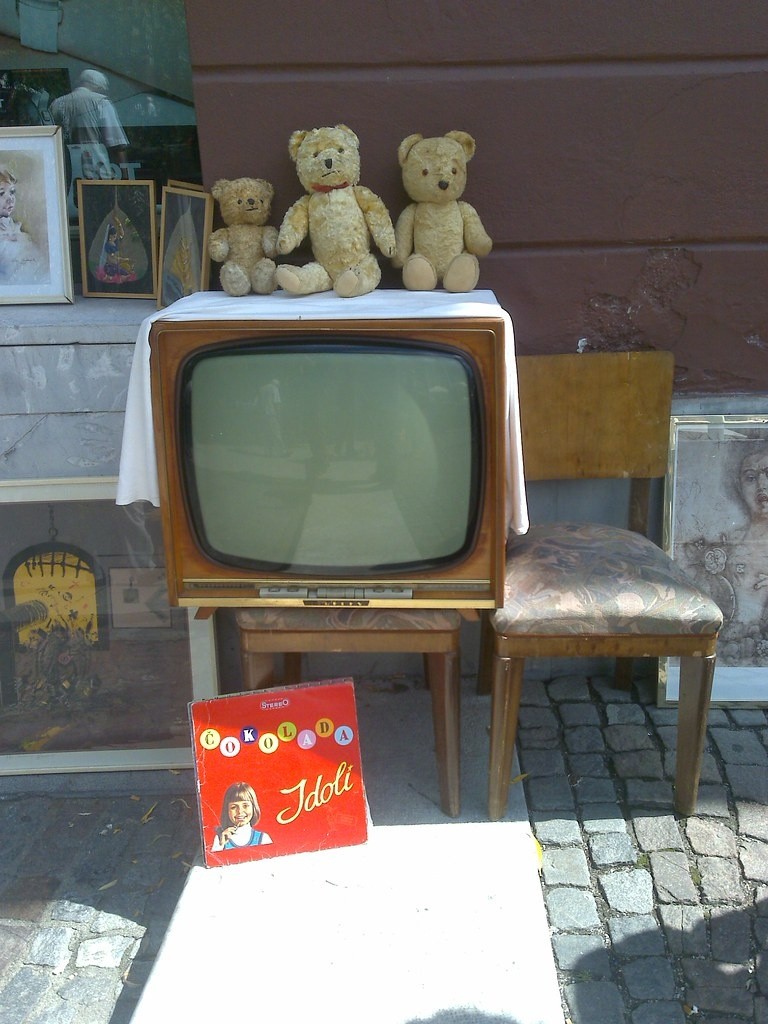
210;781;273;852
49;69;131;195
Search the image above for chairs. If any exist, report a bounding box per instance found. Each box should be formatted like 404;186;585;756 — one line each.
483;349;722;821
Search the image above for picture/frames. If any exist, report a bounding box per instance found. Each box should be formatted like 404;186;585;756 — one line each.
656;413;768;710
0;124;75;305
77;178;159;299
157;187;214;310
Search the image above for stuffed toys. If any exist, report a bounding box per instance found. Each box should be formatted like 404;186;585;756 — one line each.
273;123;397;298
207;176;279;296
392;129;493;292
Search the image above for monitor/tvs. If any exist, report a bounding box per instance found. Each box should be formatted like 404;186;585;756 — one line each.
148;291;509;612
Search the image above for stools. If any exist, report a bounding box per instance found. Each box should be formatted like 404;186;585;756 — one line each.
235;604;466;818
